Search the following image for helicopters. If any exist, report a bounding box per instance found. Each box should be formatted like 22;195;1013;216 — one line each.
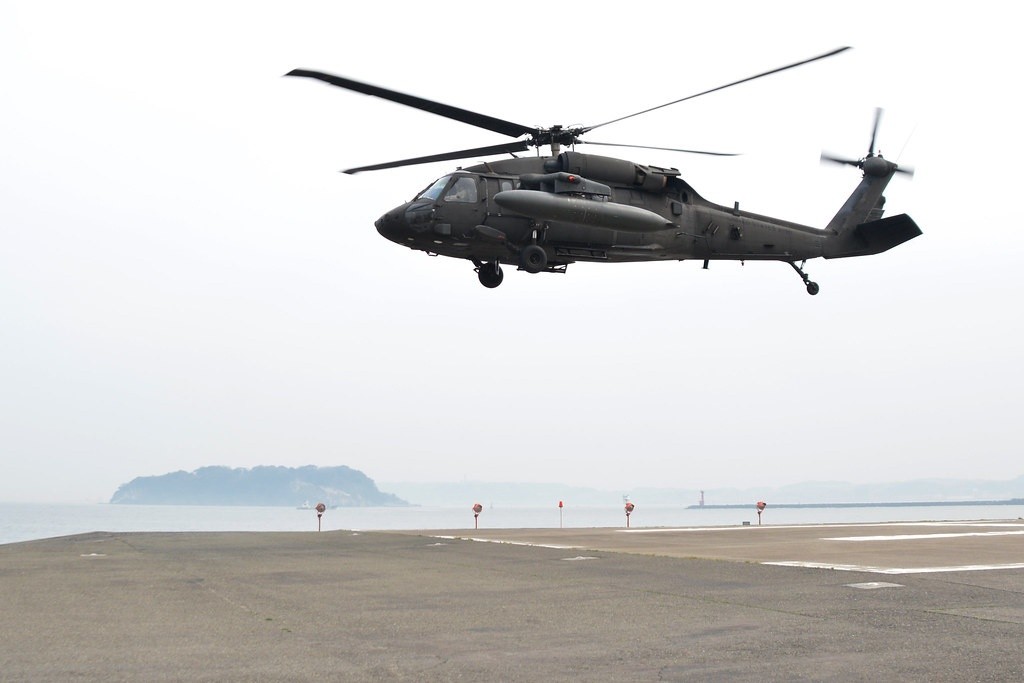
281;44;925;297
